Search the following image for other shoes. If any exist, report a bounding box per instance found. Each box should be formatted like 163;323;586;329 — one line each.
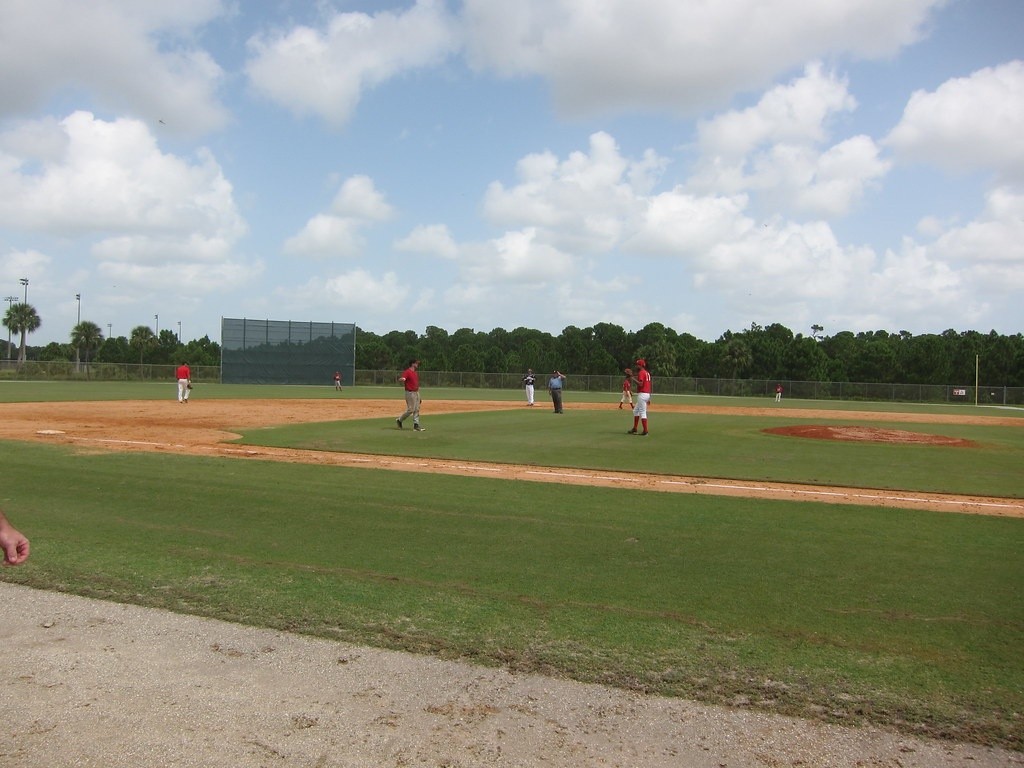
396;419;402;429
560;410;563;414
180;402;182;403
639;431;649;435
625;429;637;433
336;387;338;390
553;411;558;413
413;425;425;432
527;404;534;406
185;399;187;403
339;387;342;391
619;406;623;410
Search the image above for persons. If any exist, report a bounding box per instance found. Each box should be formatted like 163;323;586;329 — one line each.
334;371;342;391
0;509;31;568
548;370;566;414
628;360;651;436
396;358;425;431
619;377;635;409
775;384;783;402
176;360;191;404
521;369;539;406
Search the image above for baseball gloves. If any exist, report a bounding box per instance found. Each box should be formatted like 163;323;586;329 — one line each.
624;368;634;379
187;383;194;390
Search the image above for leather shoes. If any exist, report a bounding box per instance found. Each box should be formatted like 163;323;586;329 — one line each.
551;388;561;390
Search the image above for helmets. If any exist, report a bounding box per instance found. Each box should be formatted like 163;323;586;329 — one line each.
636;360;646;367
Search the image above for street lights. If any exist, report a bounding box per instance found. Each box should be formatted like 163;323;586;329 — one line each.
154;314;158;337
107;324;111;338
4;296;19;359
178;322;181;341
18;277;28;360
75;294;80;371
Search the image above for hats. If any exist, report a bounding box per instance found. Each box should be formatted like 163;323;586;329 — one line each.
528;369;532;371
553;370;558;374
409;360;420;366
182;361;189;365
336;372;339;373
